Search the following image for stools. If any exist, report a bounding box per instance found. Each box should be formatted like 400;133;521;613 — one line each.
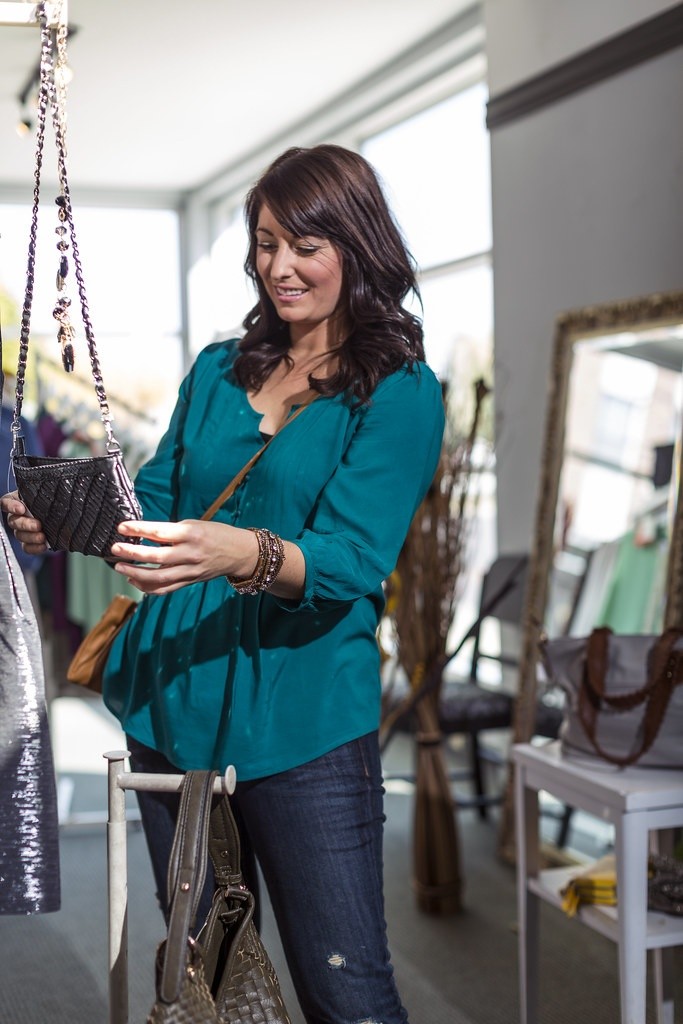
48;692;142;829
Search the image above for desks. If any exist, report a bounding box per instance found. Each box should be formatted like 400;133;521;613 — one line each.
512;739;682;1024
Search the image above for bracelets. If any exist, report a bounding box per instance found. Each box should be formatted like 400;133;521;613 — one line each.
227;526;284;596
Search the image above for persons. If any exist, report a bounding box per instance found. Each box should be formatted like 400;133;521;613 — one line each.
1;144;445;1024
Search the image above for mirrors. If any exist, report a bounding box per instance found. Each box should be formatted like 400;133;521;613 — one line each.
498;288;682;886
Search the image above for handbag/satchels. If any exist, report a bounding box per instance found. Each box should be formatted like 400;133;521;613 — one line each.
145;770;292;1024
535;623;683;770
9;453;143;562
558;842;683;927
65;594;139;694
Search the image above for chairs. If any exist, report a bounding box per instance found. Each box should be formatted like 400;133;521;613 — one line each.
374;554;523;842
535;702;576;843
